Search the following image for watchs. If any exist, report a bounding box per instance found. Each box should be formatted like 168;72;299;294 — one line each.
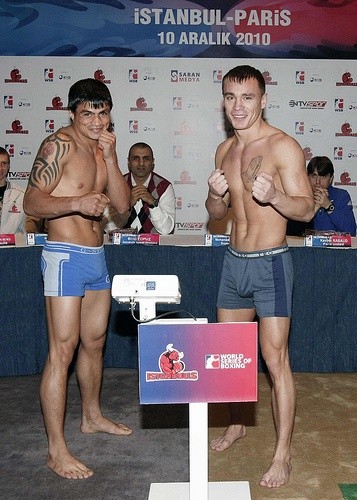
325;203;335;211
148;199;158;209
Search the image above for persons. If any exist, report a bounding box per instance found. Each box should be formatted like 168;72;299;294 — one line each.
23;79;132;480
204;66;314;488
0;147;30;236
103;142;176;235
286;156;357;238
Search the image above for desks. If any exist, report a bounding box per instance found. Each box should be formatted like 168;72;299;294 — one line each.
0;235;357;373
